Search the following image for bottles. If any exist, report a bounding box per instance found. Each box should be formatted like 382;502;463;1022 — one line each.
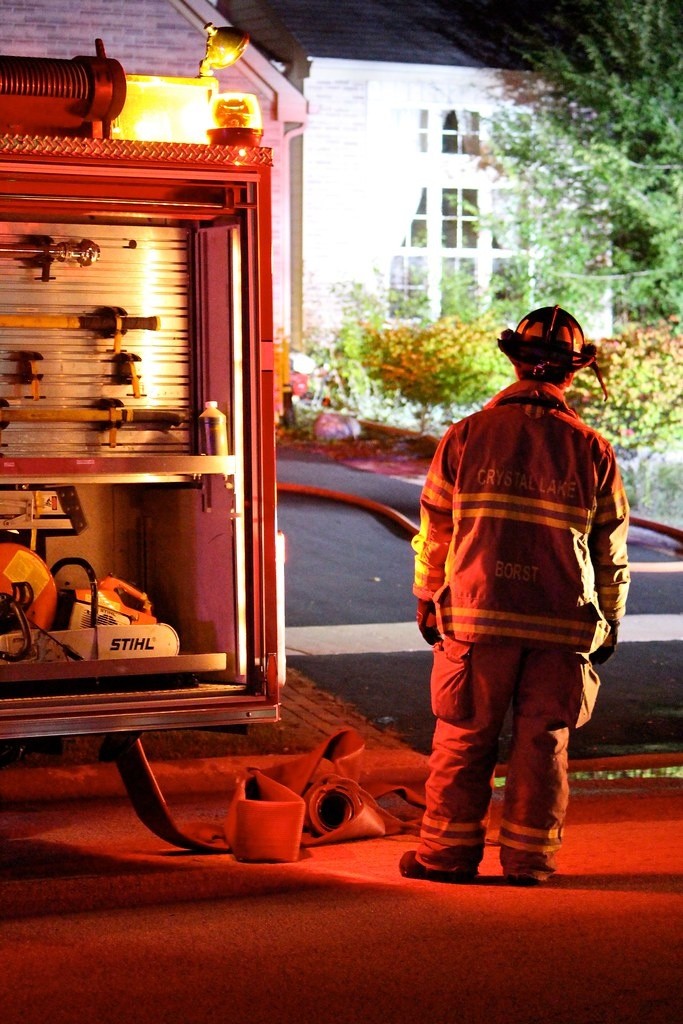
197;400;230;457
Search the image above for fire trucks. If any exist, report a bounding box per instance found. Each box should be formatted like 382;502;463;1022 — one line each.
0;25;288;746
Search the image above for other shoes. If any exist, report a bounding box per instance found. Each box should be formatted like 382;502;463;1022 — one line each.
507;874;541;886
399;851;477;884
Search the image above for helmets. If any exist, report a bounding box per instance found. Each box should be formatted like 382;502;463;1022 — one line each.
497;304;596;372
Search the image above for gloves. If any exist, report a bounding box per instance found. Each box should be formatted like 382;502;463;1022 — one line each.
416;598;444;646
590;621;620;665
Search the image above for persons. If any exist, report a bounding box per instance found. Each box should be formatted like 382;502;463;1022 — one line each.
398;306;632;887
